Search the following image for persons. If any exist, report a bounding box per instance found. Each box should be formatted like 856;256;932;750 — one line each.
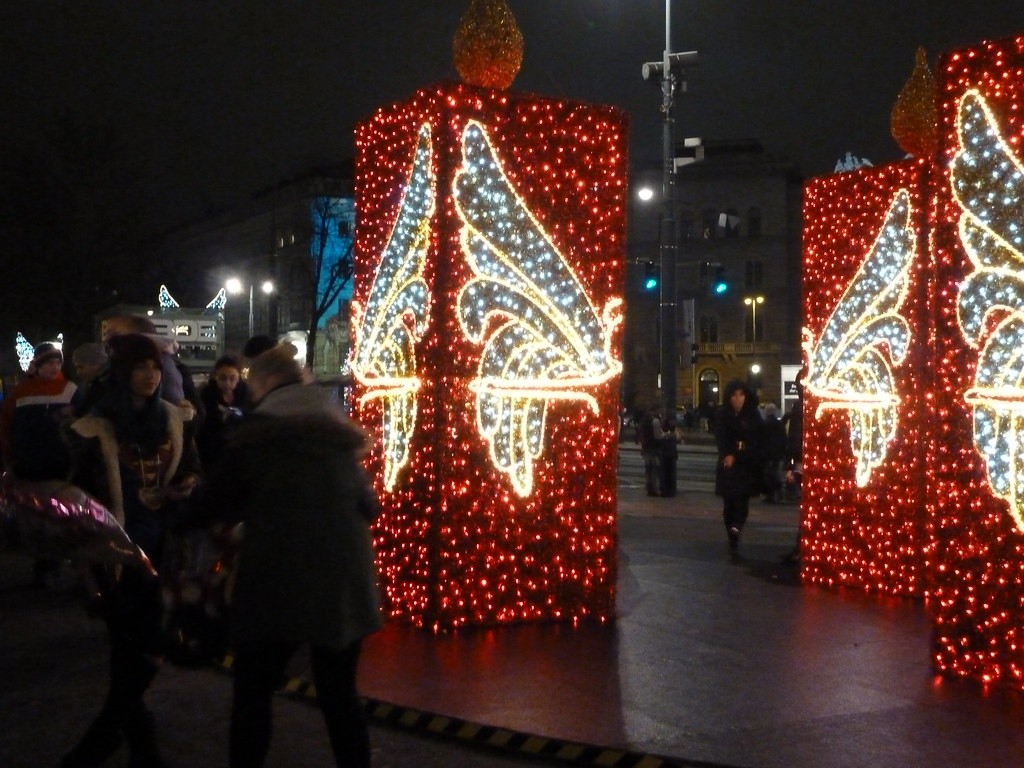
617;370;802;555
0;313;387;768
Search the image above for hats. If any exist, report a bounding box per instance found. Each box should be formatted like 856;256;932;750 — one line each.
244;335;275;356
109;334;162;375
73;343;107;366
34;344;62;368
248;343;304;402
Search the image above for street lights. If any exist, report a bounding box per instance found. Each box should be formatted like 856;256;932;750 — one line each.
744;296;765;343
221;273;276;340
